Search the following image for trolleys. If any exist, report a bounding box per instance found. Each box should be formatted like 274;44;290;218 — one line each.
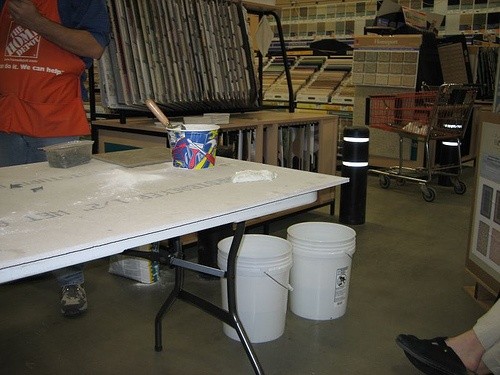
365;81;489;203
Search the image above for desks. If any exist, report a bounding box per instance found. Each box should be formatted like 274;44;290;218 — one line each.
0;146;349;375
91;110;340;270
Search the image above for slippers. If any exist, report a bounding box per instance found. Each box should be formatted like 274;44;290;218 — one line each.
404;350;446;375
396;332;466;375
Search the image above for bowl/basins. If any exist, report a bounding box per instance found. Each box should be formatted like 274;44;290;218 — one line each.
38;140;94;168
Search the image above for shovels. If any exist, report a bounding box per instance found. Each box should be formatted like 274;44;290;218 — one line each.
146;100;186;130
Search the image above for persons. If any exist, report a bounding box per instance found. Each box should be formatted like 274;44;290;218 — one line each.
396;298;500;375
0;0;114;317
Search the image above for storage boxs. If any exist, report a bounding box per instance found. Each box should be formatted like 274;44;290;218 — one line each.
43;139;94;168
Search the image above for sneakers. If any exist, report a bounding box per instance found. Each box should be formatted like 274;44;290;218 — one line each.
60;285;89;316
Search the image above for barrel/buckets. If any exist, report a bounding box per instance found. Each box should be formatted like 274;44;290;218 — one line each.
166;124;220;169
287;222;356;320
217;234;293;343
